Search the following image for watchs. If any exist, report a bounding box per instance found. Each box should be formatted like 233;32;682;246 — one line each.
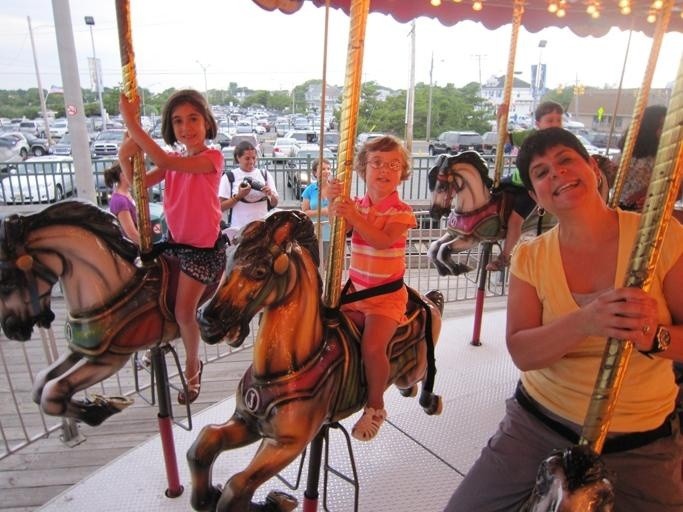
638;324;670;359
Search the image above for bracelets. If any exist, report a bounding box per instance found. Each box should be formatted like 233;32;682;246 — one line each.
232;194;239;202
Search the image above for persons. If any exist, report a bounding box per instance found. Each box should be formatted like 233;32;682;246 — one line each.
443;126;683;512
218;141;279;231
301;159;331;271
328;137;417;440
486;101;562;271
611;105;667;211
119;89;225;404
104;165;139;243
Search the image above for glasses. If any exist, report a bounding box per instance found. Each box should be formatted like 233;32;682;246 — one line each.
362;157;405;172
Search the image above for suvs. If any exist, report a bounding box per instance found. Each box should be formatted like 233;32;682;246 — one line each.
427;131;486;157
287;143;341;199
480;132;512;161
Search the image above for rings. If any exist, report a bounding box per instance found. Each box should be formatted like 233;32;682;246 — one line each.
642;324;650;333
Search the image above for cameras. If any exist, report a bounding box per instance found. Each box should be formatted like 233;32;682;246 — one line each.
242;177;264;191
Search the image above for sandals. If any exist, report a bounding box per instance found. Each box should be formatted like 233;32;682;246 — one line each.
350;404;387;442
134;341;176;370
177;359;204;405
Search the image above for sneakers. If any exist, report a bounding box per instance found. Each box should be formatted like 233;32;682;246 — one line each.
485;255;512;272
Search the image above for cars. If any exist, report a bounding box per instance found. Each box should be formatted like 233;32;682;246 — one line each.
514;120;623;155
91;158;124;201
0;156;74;202
